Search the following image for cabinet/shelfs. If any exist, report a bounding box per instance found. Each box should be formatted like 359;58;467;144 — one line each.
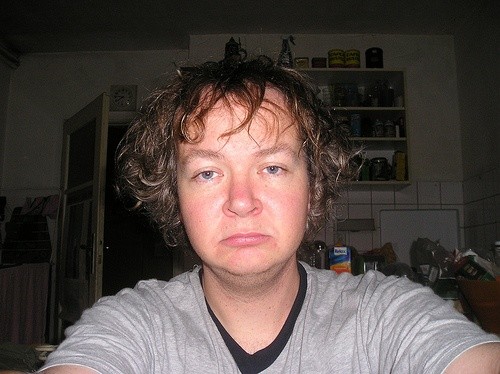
288;68;412;192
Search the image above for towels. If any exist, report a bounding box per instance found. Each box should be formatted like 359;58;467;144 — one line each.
20;194;60;220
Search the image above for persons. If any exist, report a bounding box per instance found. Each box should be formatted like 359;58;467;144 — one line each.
36;42;500;374
